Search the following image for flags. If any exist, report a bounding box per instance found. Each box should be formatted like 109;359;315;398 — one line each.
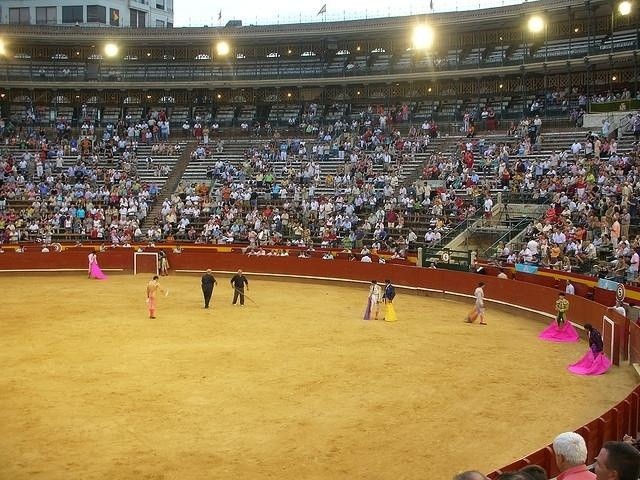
218;11;222;20
113;11;119;21
317;5;327;16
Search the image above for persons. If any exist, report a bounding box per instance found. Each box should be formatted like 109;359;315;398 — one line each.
1;108;289;257
88;251;98;281
467;281;489;325
582;323;604;359
554;293;570;327
158;247;169;276
593;440;640;480
520;465;547;479
623;433;639;453
493;469;536;480
381;279;398;322
454;469;488;480
552;431;598;480
229;268;249;306
288;86;640;316
201;269;218;308
146;276;169;319
362;280;382;321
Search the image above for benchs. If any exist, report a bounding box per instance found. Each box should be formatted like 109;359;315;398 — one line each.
0;116;640;285
